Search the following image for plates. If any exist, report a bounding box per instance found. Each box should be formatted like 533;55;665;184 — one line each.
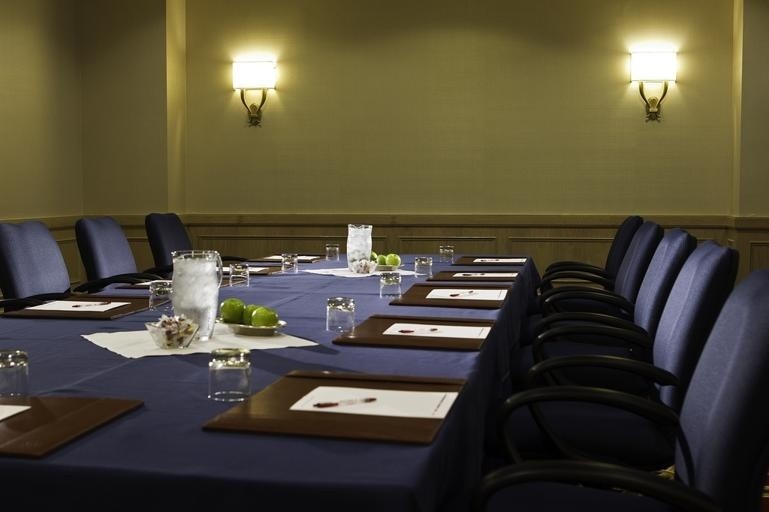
216;317;287;336
375;264;399;271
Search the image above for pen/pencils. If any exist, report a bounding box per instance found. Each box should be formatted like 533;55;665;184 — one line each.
314;398;377;407
71;299;111;308
450;291;473;296
462;273;486;276
398;328;437;333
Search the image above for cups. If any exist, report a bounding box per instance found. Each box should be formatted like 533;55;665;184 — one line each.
281;253;299;275
228;264;250;288
209;349;253;403
325;297;355;335
325;243;340;263
345;224;373;271
438;244;455;264
0;351;30;405
148;280;173;314
170;250;225;341
413;257;434;279
379;271;402;300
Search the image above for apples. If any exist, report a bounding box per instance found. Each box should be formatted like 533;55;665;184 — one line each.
386;253;401;266
251;306;279;326
220;298;246;327
376;255;386;265
371;251;377;263
241;303;259;326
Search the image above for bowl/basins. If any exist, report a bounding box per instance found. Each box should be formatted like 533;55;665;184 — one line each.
145;320;201;351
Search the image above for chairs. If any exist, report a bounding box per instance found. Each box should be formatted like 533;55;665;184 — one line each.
1;213;246;317
